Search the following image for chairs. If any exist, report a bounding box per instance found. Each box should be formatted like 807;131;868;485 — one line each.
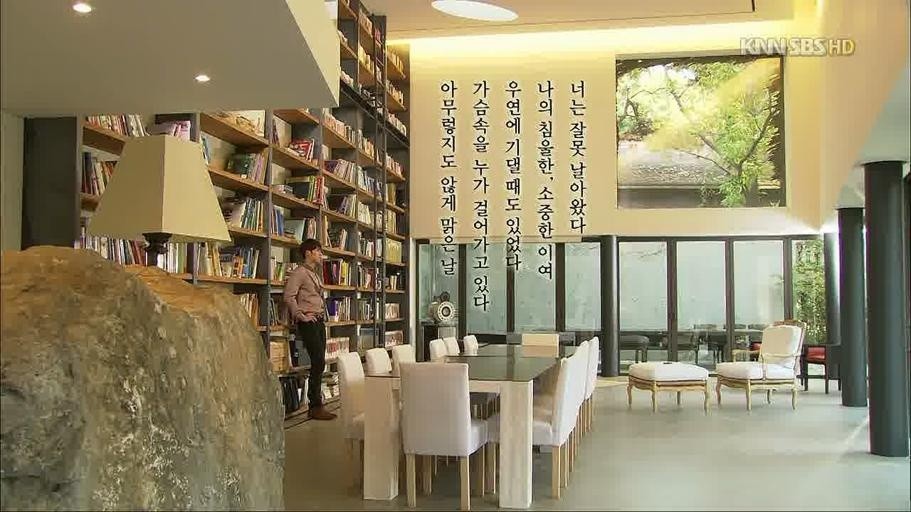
336;332;600;512
677;319;841;413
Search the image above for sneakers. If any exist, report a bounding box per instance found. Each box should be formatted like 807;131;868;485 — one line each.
307;408;338;420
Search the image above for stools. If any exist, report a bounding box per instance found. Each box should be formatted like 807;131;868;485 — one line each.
626;361;711;417
618;334;650;363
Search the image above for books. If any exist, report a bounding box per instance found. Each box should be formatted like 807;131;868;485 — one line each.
82;0;406;413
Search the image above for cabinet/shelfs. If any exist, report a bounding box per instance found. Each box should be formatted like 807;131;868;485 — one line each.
421;320;458;361
18;0;410;422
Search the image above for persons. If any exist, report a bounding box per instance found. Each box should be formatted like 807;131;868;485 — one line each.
282;238;339;421
